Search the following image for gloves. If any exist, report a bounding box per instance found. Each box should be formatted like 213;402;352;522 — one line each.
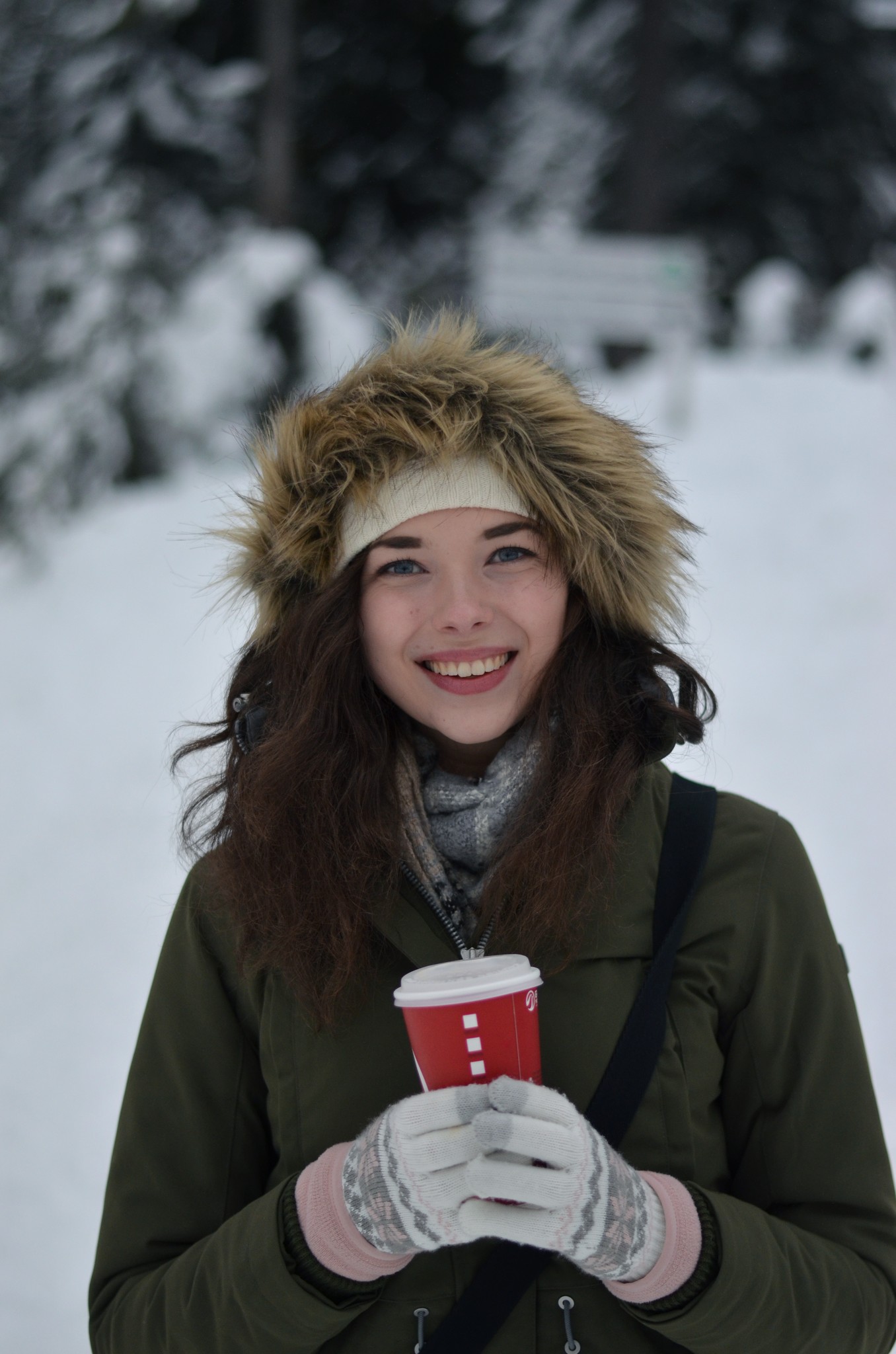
342;1081;537;1256
457;1072;662;1284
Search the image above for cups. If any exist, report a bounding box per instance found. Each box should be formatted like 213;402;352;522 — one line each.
392;950;538;1108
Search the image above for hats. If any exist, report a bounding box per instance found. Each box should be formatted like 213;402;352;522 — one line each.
335;451;543;575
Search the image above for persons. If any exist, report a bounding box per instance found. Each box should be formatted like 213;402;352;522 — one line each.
89;303;896;1354
